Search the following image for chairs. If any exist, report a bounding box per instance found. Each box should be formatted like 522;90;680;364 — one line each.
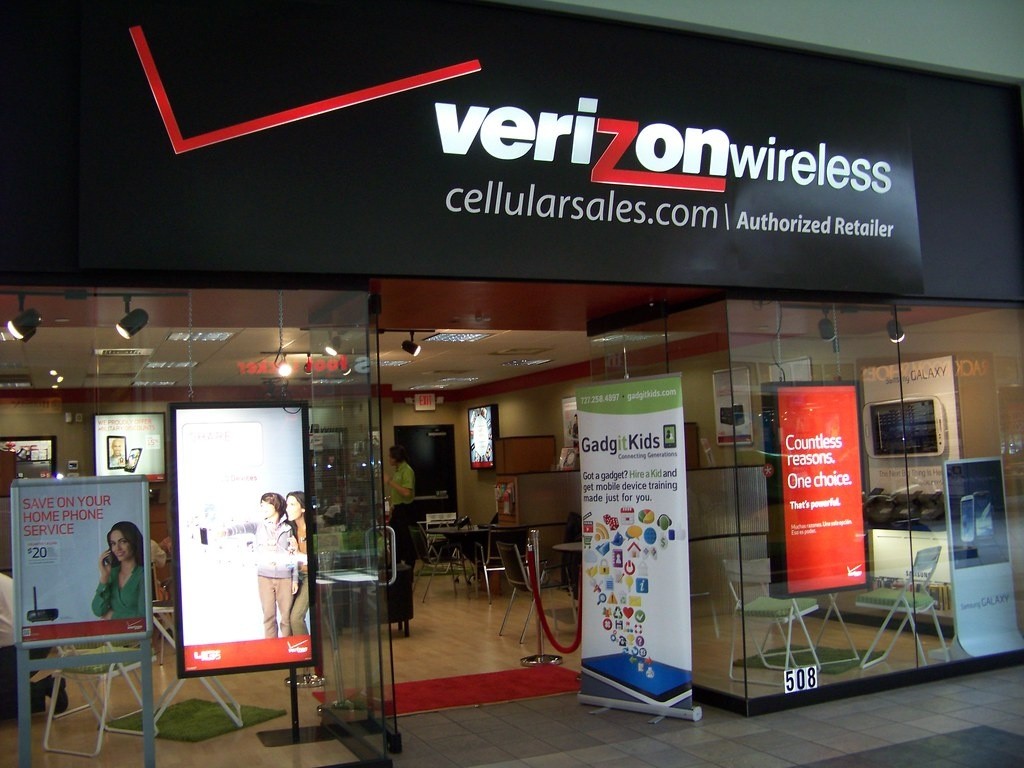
722;554;822;688
411;518;572;605
379;564;414;639
42;640;161;759
496;540;578;644
855;546;951;667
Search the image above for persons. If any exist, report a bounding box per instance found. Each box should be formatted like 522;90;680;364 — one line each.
110;440;125;458
569;414;579;447
382;444;419;570
281;490;312;635
149;533;172;600
91;520;146;618
0;571;68;720
218;492;299;639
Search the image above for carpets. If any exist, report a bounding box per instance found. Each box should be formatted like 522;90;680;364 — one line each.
312;662;585;725
99;696;286;743
734;644;886;675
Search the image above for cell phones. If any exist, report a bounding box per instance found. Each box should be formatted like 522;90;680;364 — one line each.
105;552;113;565
106;435;143;473
863;395;944;458
973;491;995;537
960;496;976;542
200;527;207;545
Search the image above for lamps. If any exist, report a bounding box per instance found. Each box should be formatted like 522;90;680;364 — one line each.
818;308;836;342
116;296;149;340
886;311;905;343
325;336;342;357
8;294;43;344
402;332;421;357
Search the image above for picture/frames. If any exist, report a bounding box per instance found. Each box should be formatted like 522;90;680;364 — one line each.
557;446;578;472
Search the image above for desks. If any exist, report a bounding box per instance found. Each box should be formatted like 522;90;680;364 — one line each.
426;526;491;586
152;605;244;731
551;542;583;553
